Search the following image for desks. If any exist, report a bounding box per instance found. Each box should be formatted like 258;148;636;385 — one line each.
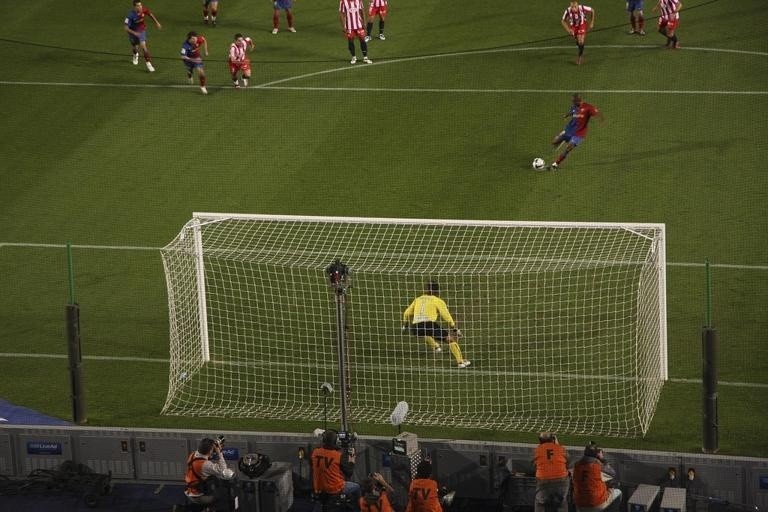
389;450;419;497
225;457;295;511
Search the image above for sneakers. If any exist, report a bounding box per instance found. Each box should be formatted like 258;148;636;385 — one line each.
350;55;358;64
627;29;646;36
362;56;373;64
364;35;372;42
187;77;194;86
575;54;582;65
212;21;216;26
199;85;208;96
665;41;680;50
131;54;140;65
455;360;472;371
288;27;296;33
433;346;442;354
271;28;279;35
378;33;386;41
204;20;209;24
551;162;559;171
145;61;156;73
233;79;249;90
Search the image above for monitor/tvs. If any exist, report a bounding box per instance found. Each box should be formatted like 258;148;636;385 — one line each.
392;431;418;455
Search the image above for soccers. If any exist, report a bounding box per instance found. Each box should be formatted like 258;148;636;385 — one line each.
532;158;545;170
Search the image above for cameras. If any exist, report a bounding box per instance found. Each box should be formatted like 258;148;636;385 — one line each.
552;433;556;439
594;449;603;457
213;434;225;450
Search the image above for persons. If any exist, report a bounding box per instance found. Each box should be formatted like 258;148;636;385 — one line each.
337;0;373;65
227;33;255;87
530;429;571;511
178;31;210;96
571;441;623;511
559;0;596;65
363;0;389;43
357;470;395;512
123;0;162;75
310;428;361;502
269;0;297;35
651;0;682;49
201;0;219;27
182;437;231;504
401;280;471;368
550;93;604;167
623;0;647;37
405;461;443;511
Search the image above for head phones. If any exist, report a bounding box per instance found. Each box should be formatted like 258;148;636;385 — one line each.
321;429;339;444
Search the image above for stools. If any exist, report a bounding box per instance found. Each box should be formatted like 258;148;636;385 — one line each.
314;494;352;511
653;482;690;510
624;477;659;512
